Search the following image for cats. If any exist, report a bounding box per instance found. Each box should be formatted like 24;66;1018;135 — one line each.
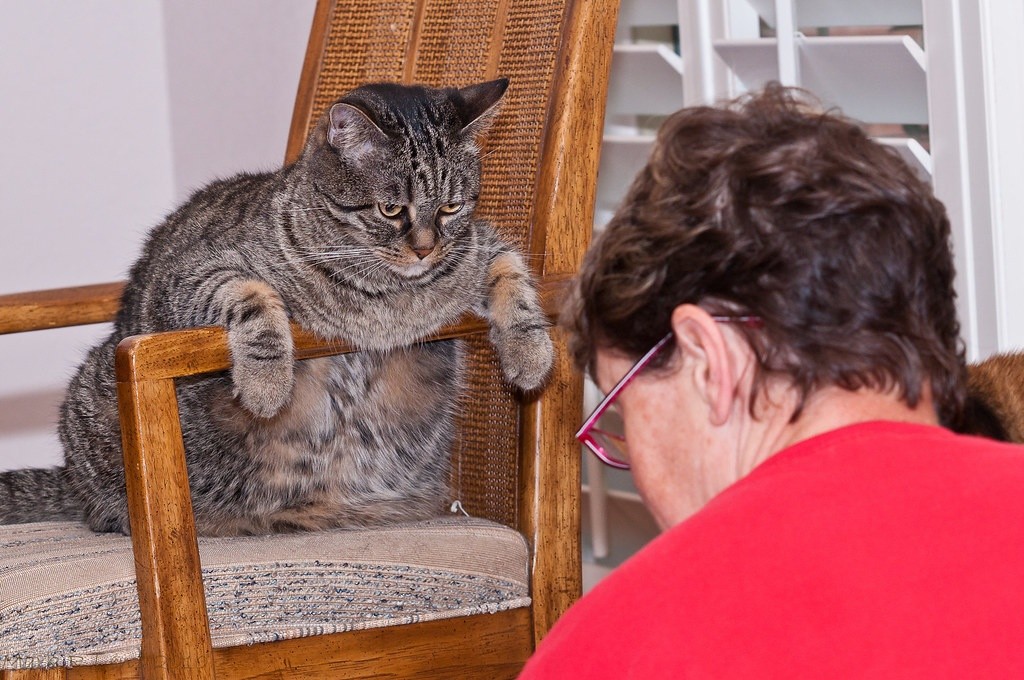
0;76;555;538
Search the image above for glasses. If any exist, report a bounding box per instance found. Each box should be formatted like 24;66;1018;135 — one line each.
573;314;770;470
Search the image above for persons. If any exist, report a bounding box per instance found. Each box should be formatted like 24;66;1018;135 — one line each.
514;81;1024;680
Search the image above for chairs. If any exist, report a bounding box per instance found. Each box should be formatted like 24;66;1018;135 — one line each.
0;1;622;679
